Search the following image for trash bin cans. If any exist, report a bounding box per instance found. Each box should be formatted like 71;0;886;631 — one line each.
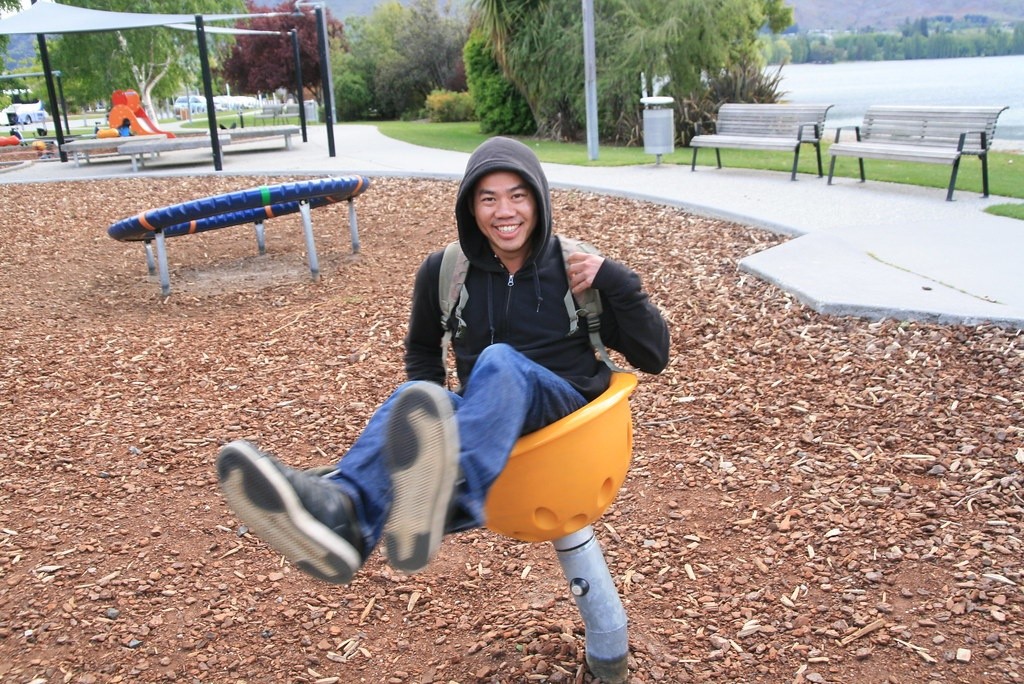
639;97;674;154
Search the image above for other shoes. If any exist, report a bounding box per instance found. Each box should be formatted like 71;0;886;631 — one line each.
215;440;367;585
383;380;459;572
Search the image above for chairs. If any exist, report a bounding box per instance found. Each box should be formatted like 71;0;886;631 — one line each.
254;104;283;125
278;105;302;125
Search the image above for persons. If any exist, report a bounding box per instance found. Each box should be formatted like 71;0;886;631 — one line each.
11;128;27;146
115;117;134;137
217;137;670;583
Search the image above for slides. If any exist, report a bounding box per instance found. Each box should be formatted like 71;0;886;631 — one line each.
109;88;178;138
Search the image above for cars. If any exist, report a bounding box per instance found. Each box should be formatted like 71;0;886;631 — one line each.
172;95;260;120
0;102;47;126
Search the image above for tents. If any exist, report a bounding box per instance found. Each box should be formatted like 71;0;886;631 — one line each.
1;0;337;170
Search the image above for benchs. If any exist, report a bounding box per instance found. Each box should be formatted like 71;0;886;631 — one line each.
688;102;835;182
60;134;166;167
117;135;232;171
827;104;1010;201
207;124;299;159
